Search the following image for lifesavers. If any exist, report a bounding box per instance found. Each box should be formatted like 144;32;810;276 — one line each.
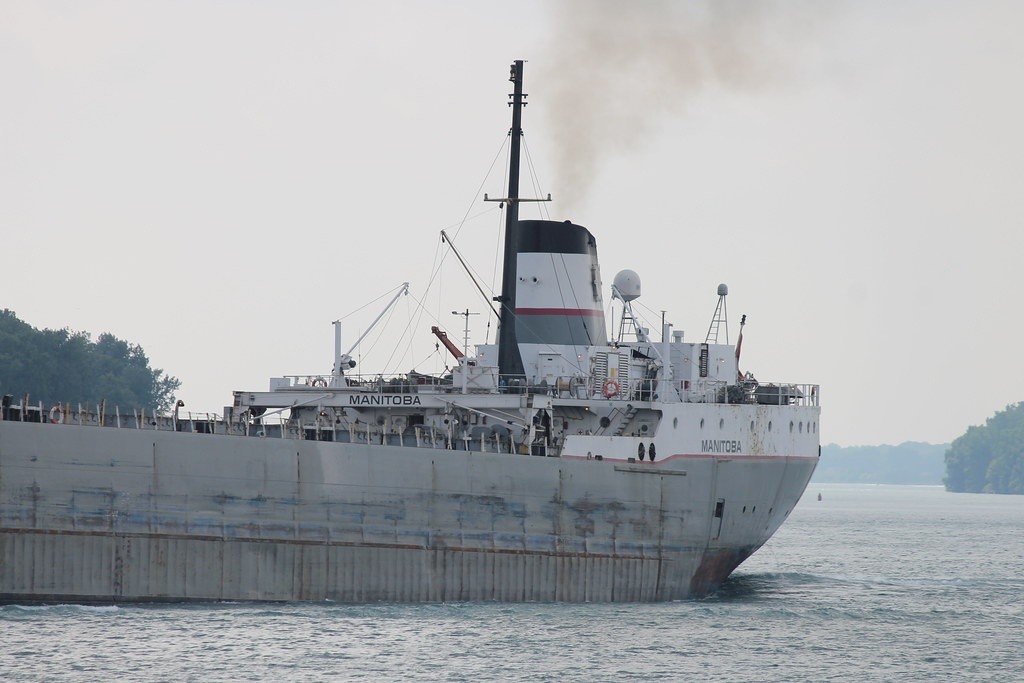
603;381;619;396
49;405;64;423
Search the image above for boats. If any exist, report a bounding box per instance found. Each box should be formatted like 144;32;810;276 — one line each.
0;63;823;608
